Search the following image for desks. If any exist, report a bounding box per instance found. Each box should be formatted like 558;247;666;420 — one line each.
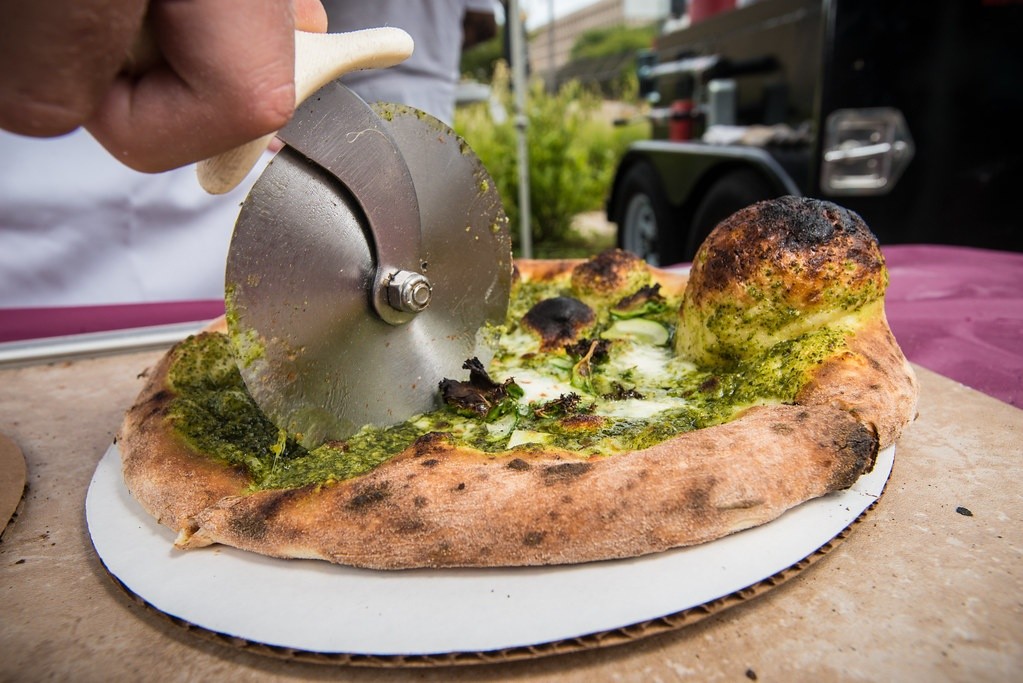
0;242;1022;413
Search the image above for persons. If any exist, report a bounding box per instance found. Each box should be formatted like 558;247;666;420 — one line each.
1;0;466;350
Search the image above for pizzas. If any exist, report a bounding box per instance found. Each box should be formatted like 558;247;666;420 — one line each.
114;196;920;574
